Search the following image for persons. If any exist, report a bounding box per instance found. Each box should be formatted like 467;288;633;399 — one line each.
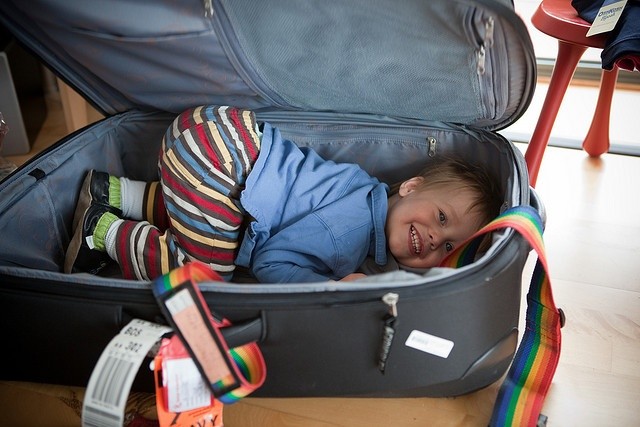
65;105;502;284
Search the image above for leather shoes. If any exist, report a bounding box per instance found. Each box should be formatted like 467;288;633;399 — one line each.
72;168;121;236
64;205;119;277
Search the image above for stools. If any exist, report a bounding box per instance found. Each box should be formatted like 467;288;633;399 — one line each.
524;2;640;191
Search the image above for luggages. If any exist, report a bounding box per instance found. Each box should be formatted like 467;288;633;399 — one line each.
0;0;564;425
